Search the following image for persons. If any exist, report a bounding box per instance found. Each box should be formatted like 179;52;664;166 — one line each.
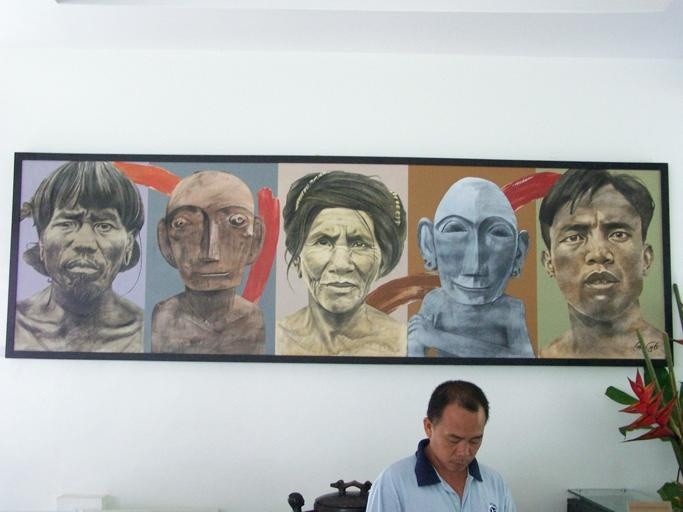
274;171;407;358
536;168;666;360
366;380;519;512
150;170;266;354
407;176;536;358
14;161;144;353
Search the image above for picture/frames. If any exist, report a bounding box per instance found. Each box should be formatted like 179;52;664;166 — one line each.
4;152;675;368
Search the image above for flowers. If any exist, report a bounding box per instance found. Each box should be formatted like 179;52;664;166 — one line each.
605;283;683;512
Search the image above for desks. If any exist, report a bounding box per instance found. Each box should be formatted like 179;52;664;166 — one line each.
567;487;683;512
